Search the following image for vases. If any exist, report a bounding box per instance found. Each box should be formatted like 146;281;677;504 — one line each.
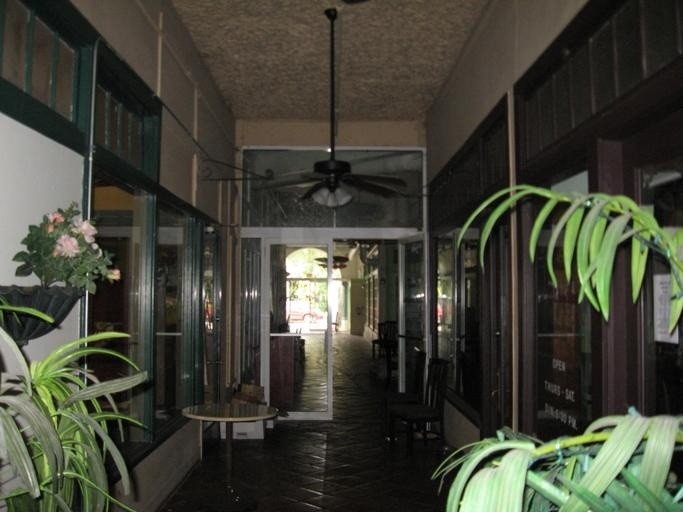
0;281;90;361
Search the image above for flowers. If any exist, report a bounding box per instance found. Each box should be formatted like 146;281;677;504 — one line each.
2;195;122;301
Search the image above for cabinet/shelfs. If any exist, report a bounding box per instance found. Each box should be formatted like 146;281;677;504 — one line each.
254;330;307;412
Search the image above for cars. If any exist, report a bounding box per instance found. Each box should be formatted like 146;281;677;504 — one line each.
286;301;325;324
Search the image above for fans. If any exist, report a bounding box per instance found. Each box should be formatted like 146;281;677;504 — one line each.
242;5;410;200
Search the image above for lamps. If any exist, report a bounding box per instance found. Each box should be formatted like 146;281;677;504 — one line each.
302;184;354;210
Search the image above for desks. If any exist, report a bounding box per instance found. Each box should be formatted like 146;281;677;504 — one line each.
164;401;282;510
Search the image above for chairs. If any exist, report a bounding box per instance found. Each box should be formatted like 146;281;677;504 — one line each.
367;315;452;466
